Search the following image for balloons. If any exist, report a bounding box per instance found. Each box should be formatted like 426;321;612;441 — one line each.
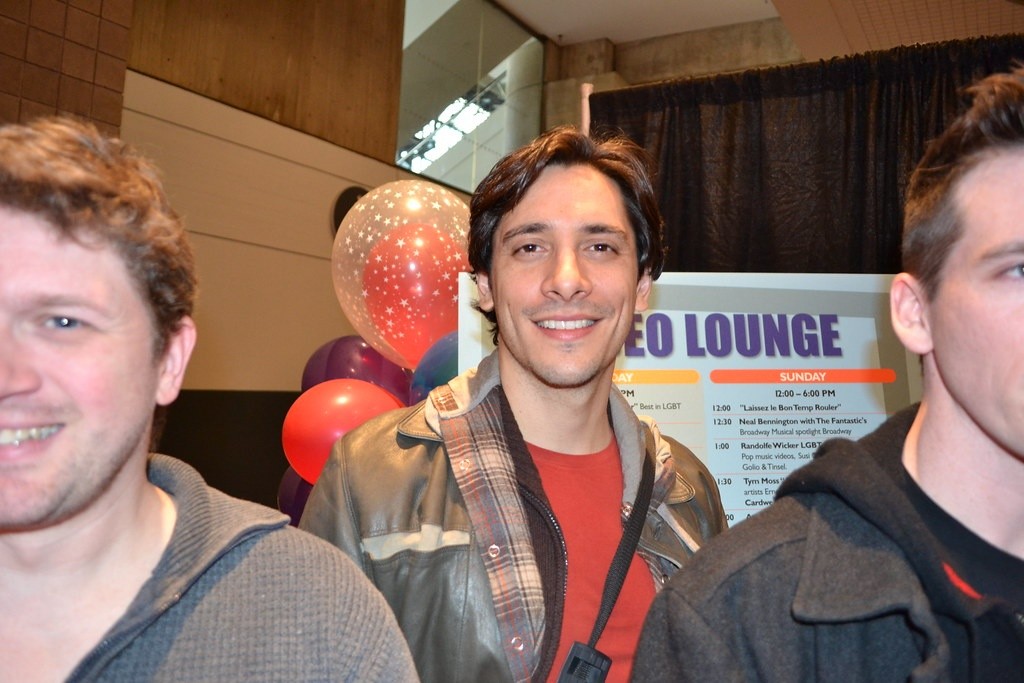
281;379;405;485
414;330;459;400
364;224;472;368
335;179;473;371
276;466;314;528
300;335;412;407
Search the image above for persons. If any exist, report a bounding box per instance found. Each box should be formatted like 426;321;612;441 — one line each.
626;63;1024;683
297;127;729;683
1;108;420;683
